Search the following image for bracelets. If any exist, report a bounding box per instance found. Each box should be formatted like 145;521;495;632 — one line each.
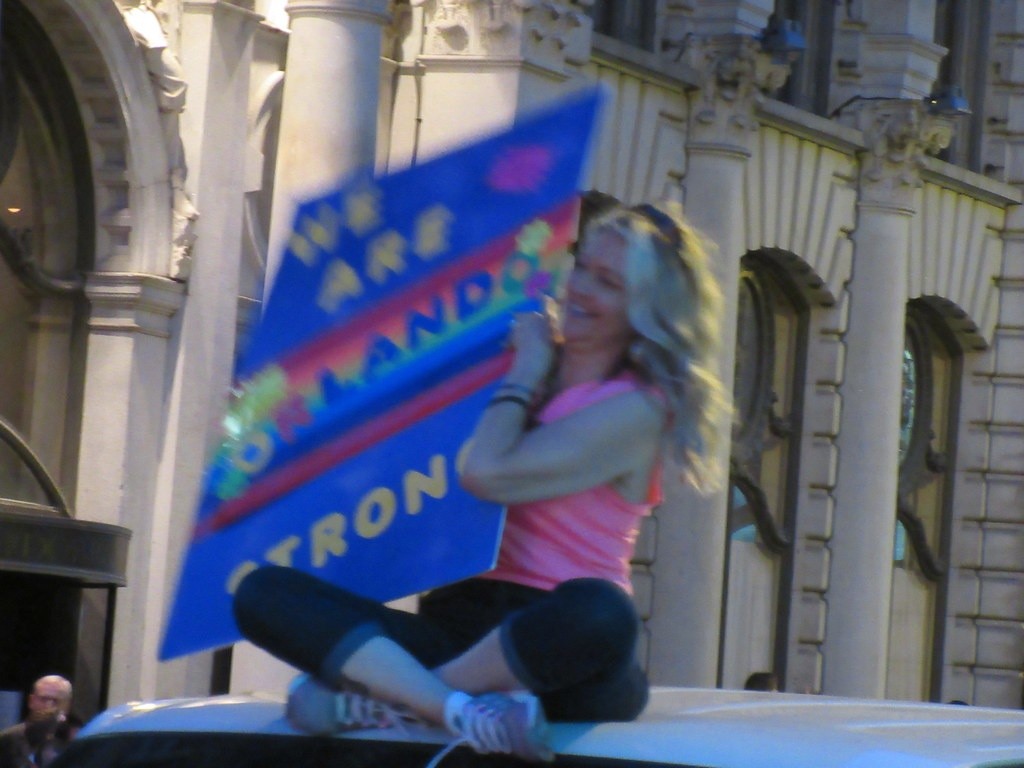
480;383;538;418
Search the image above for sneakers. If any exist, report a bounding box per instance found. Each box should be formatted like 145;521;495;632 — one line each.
429;689;553;767
285;673;425;742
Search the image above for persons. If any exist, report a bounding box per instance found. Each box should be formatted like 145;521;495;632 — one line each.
0;675;74;768
234;198;735;758
